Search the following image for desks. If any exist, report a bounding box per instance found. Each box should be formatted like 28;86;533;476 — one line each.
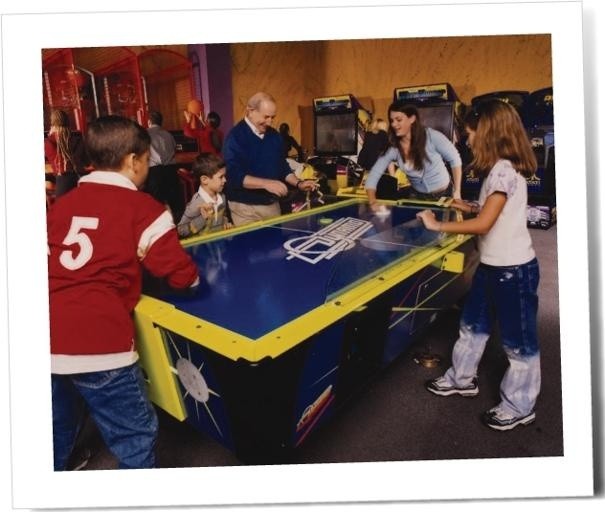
132;198;475;464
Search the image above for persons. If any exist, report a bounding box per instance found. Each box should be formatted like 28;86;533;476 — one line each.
143;110;184;226
278;123;303;164
413;97;542;431
183;110;226;158
364;100;463;216
44;109;94;200
47;113;199;471
178;151;232;240
222;90;319;228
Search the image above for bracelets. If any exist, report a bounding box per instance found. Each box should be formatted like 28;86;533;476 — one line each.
297;180;302;189
439;221;444;232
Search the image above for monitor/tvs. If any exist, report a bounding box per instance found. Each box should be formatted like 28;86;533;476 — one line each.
415;100;455;144
314;111;358;157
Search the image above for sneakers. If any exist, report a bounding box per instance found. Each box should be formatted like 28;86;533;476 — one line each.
481;404;538;431
425;374;482;399
66;445;93;471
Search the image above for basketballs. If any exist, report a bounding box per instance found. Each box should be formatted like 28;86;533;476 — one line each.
187;99;204;116
